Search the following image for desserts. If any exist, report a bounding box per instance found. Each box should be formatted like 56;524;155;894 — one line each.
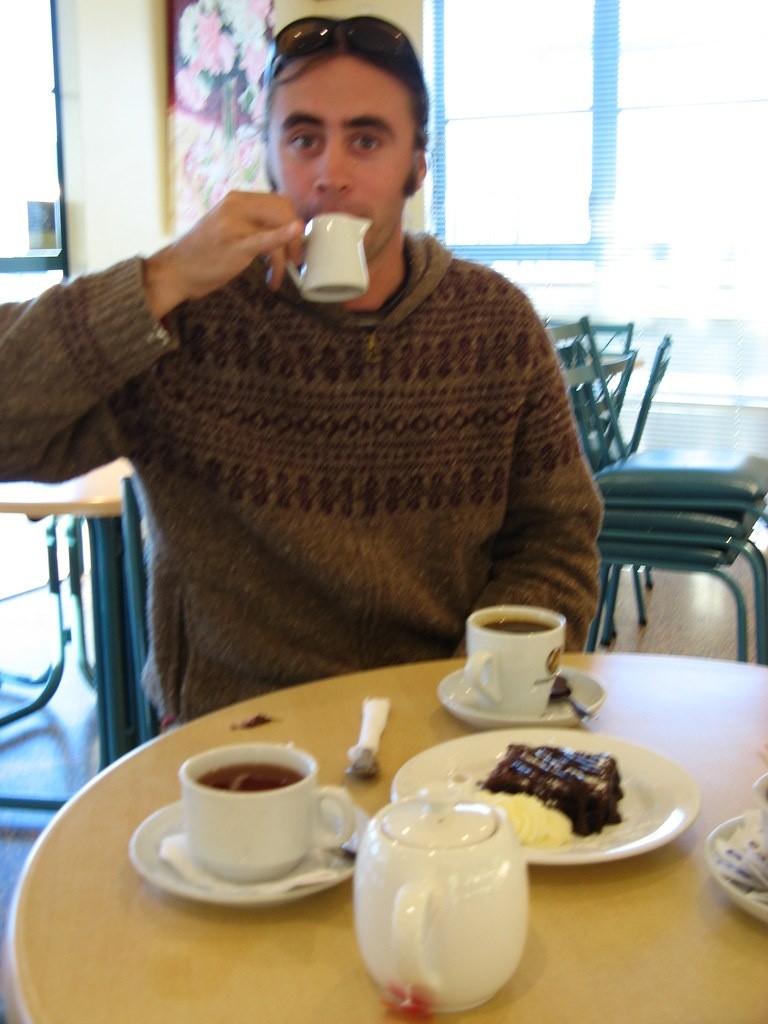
464;792;570;845
486;746;621;836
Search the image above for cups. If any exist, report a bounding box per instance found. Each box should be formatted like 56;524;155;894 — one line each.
178;743;356;881
465;606;567;719
752;772;768;853
284;213;372;304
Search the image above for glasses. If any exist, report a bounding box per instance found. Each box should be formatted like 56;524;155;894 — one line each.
270;16;410;70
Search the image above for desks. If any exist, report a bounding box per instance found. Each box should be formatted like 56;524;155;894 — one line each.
10;650;768;1024
0;459;137;808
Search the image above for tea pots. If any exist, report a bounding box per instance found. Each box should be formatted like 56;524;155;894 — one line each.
351;786;529;1010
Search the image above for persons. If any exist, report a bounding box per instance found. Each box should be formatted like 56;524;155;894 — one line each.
1;16;605;735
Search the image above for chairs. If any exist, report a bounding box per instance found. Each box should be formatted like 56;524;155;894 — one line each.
0;320;768;809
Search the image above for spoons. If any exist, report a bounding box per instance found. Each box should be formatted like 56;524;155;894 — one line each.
549;675;596;718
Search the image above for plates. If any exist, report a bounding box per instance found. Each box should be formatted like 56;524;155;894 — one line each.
706;809;768;923
130;800;371;905
392;726;703;867
436;669;608;731
